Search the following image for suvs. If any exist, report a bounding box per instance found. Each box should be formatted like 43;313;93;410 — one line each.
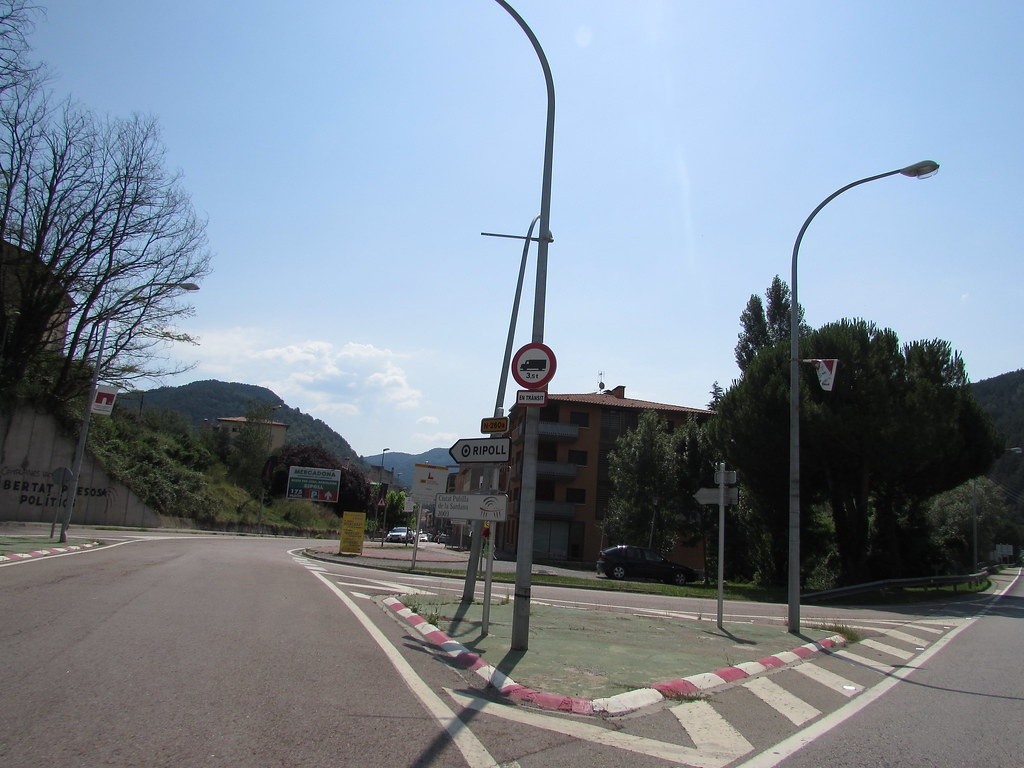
596;545;699;586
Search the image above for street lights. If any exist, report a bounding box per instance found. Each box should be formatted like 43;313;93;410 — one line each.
256;405;282;533
59;281;202;542
787;160;941;635
972;446;1022;574
454;214;555;603
371;448;390;543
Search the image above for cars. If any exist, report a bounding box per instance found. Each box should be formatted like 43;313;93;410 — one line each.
387;526;415;544
414;530;451;544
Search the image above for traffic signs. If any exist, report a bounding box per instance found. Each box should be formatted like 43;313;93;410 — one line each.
449;438;512;464
286;466;341;504
